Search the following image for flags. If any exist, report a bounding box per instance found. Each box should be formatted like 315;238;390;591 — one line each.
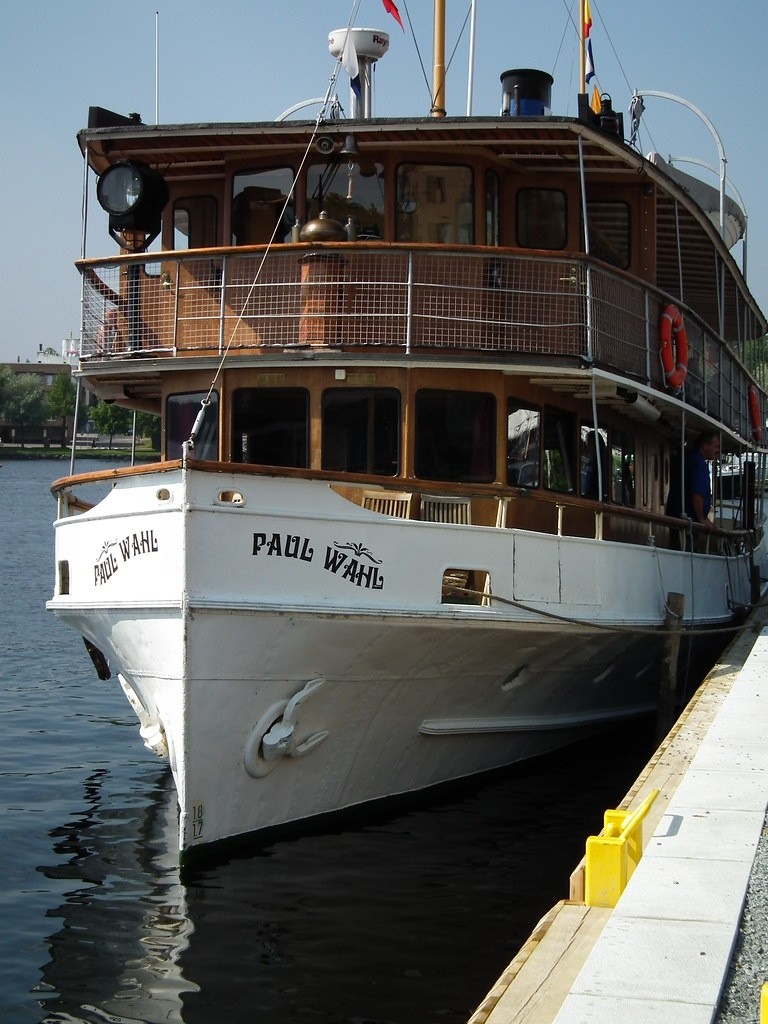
384;0;407;36
584;0;595;81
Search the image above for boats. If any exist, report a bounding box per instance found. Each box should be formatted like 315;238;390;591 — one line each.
47;0;767;852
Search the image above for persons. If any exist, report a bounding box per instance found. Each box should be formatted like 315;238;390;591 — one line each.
663;433;721;528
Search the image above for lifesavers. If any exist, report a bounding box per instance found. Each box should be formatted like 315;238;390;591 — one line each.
661;302;692;392
749;381;764;445
